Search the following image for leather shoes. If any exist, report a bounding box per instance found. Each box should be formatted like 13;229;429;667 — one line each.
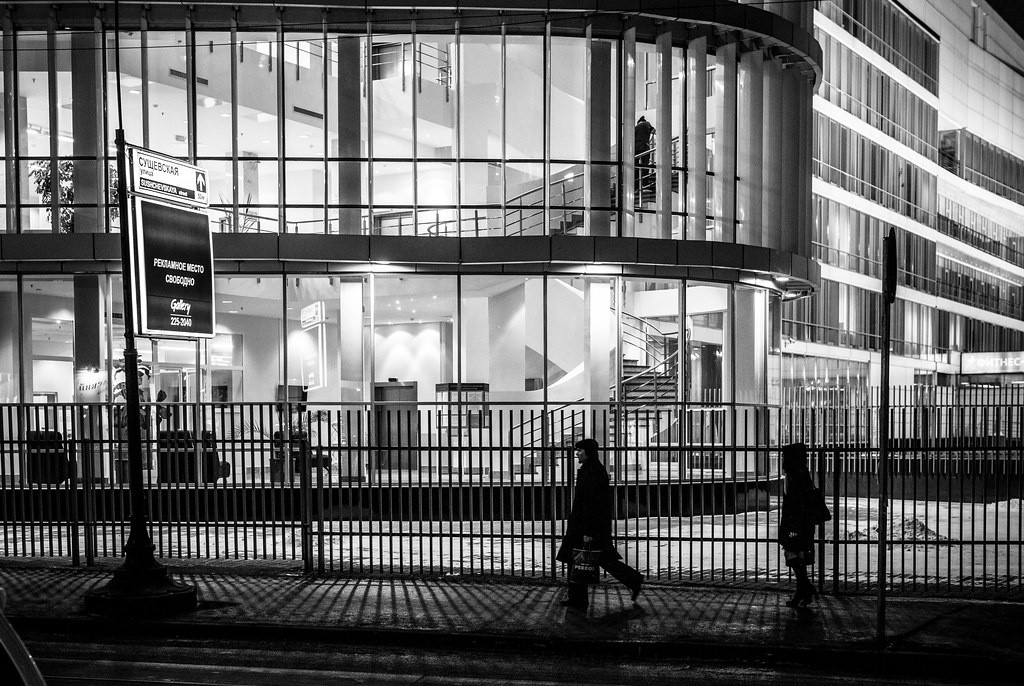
629;571;644;600
560;598;590;609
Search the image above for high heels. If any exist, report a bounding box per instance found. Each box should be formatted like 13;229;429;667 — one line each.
800;583;816;607
785;592;803;607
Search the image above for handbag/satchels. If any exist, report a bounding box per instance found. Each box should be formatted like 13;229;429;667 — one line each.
570;547;601;586
810;486;831;521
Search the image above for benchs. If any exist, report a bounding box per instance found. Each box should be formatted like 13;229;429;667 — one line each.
159;429;220;483
26;431;77;489
274;431;332;478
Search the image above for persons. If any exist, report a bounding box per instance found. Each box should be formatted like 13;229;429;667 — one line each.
777;442;819;608
554;438;647;621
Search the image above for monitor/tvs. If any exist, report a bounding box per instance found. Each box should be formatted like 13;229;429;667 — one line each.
278;385;308;412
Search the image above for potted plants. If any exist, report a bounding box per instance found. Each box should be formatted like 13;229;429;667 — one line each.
30;161;131;233
106;349;170;484
